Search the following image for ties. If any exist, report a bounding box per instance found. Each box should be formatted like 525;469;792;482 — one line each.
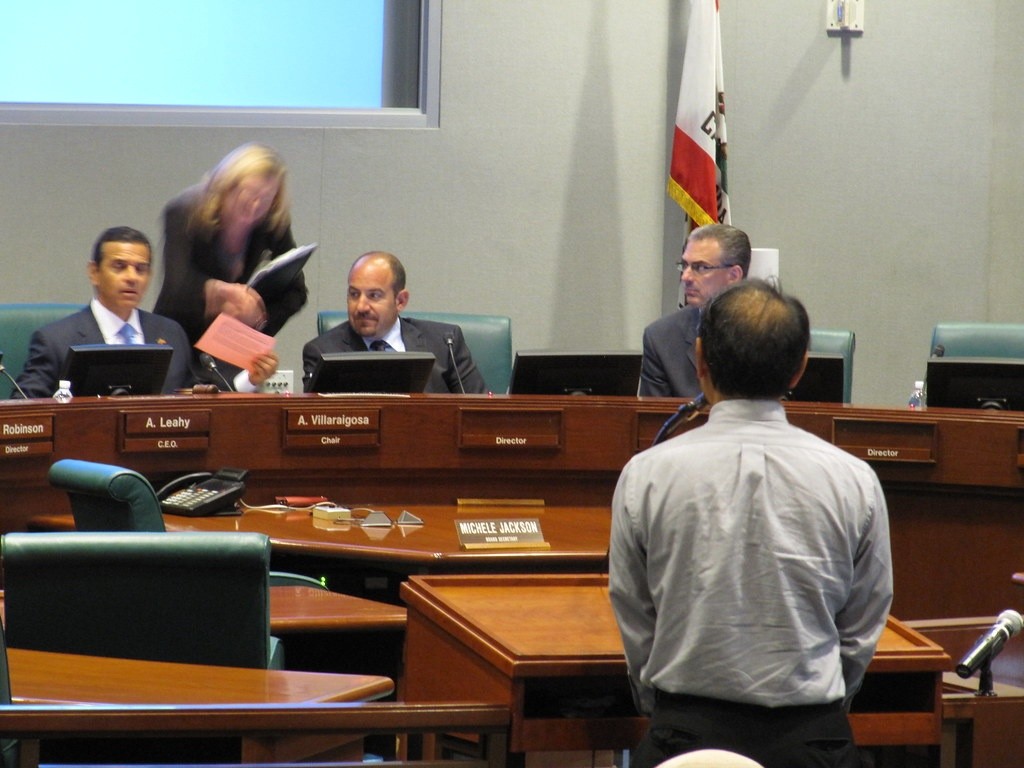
122;324;135;343
371;341;389;351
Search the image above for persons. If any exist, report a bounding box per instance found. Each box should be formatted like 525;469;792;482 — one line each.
153;141;308;367
636;223;752;396
302;251;491;394
8;226;279;397
608;282;893;768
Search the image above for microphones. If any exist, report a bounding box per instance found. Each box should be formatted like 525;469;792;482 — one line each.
443;331;465;394
199;353;233;392
956;609;1024;679
651;392;707;446
921;344;945;406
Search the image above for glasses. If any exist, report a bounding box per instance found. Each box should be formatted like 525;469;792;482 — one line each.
676;259;738;276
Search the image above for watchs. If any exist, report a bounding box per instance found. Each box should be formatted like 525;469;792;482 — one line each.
257;314;269;332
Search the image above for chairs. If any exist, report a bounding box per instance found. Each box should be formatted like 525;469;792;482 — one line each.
317;311;513;395
808;329;856;405
51;459;166;533
930;323;1024;361
1;529;285;672
0;304;90;399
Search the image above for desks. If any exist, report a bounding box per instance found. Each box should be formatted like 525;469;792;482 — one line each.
0;389;1024;691
28;504;611;569
265;584;410;634
7;646;397;765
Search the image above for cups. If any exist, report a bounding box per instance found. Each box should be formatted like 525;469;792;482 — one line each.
263;370;294;394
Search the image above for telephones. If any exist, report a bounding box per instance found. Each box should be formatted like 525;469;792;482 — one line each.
154;467;251;517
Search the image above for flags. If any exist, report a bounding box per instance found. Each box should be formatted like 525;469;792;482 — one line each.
666;0;732;313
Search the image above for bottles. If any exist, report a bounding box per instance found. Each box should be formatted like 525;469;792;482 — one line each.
908;380;924;408
53;380;73;398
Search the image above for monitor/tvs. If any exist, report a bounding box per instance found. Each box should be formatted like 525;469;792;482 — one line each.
54;344;173;396
780;352;844;403
509;349;644;397
306;351;436;394
926;357;1024;411
